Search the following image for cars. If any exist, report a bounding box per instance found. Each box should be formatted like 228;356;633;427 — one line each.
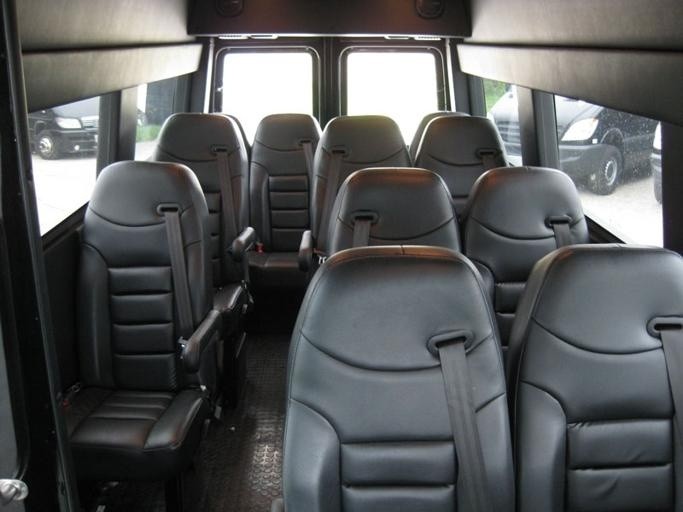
26;96;99;160
484;84;662;204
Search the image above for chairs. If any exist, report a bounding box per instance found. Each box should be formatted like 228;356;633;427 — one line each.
514;244;682;512
149;112;249;359
460;168;589;365
271;245;515;512
59;159;222;480
310;117;412;248
248;115;323;288
415;116;509;226
408;111;470;164
